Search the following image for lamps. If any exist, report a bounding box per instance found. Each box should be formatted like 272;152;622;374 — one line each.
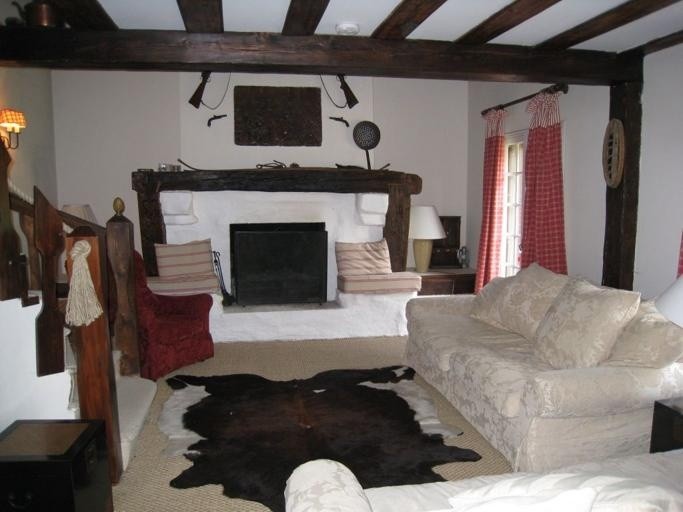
0;109;27;150
410;204;448;273
61;203;98;229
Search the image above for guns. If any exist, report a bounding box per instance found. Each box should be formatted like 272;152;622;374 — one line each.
189;72;211;108
328;116;349;127
208;114;227;127
337;74;359;109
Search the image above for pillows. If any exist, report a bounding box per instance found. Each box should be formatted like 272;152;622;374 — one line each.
468;261;642;369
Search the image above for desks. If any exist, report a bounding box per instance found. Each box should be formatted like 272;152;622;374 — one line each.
0;417;113;512
418;272;474;296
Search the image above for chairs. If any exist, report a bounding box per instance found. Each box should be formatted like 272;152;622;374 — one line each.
134;249;218;377
333;238;423;308
144;236;225;318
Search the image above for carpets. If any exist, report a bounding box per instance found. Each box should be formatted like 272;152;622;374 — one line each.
112;336;516;510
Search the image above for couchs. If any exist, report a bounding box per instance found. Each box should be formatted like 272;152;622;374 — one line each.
402;261;681;471
284;450;680;511
